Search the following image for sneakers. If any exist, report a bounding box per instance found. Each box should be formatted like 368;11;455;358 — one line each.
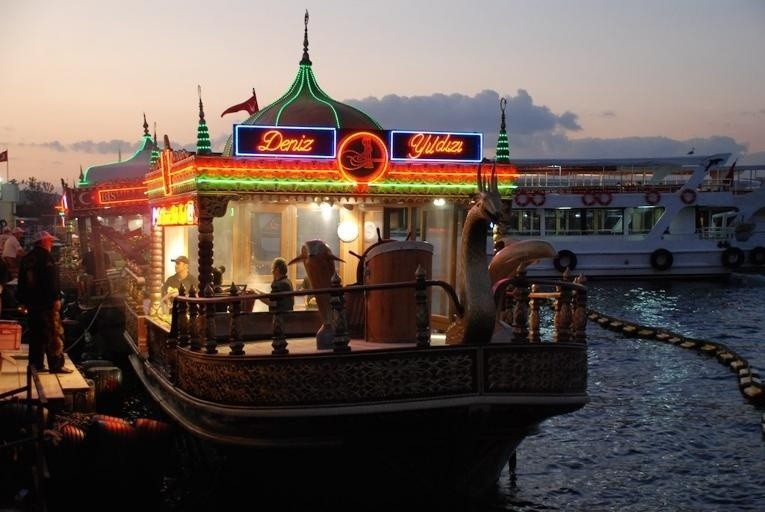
28;367;48;373
50;367;72;373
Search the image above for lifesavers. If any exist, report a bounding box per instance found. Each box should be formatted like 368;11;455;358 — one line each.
581;189;597;206
645;189;661;205
531;190;546;206
515;189;530;207
597;189;612;206
680;188;697;205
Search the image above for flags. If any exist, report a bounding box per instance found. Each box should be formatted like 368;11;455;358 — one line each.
221;95;258;116
0;151;7;162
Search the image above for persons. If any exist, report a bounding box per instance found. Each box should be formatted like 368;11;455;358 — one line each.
0;225;74;374
248;257;294;312
161;256;200;314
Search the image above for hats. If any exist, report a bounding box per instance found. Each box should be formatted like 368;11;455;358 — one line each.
12;228;23;233
35;231;54;240
172;256;188;264
